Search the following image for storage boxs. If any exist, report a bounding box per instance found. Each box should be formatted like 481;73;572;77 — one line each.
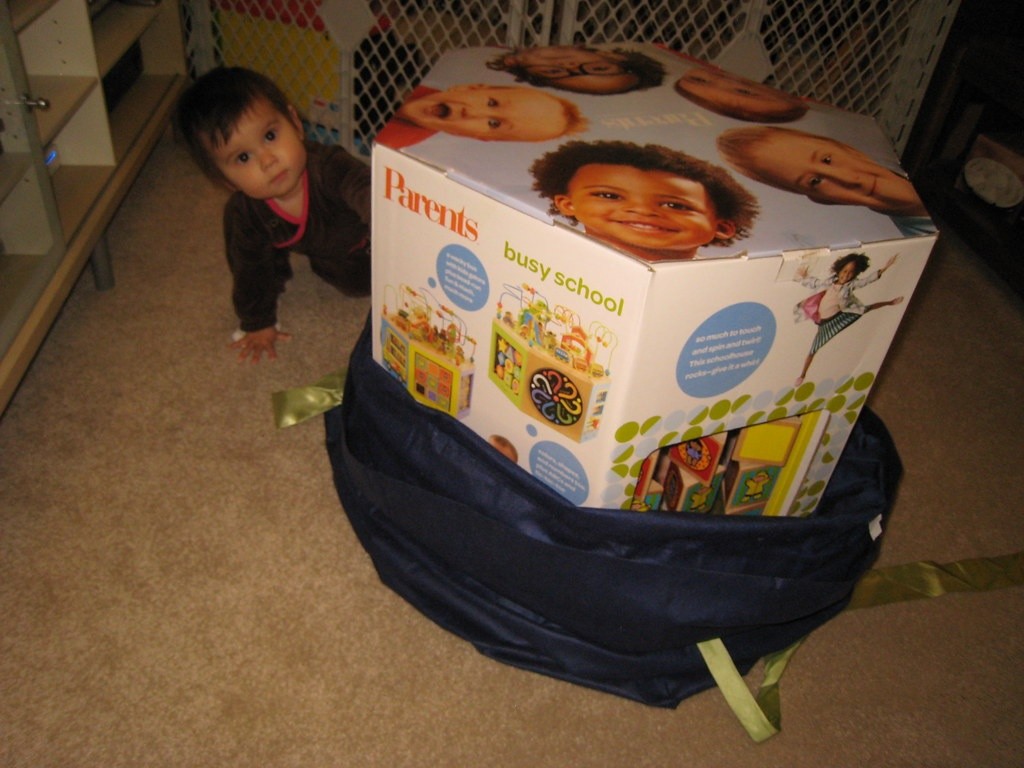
370;39;940;523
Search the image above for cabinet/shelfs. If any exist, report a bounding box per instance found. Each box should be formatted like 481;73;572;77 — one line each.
0;0;188;417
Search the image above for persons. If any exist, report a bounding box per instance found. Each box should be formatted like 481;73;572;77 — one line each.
376;83;587;152
488;435;517;463
794;252;904;386
177;68;372;364
658;42;838;122
717;126;937;238
486;44;663;96
531;140;757;264
